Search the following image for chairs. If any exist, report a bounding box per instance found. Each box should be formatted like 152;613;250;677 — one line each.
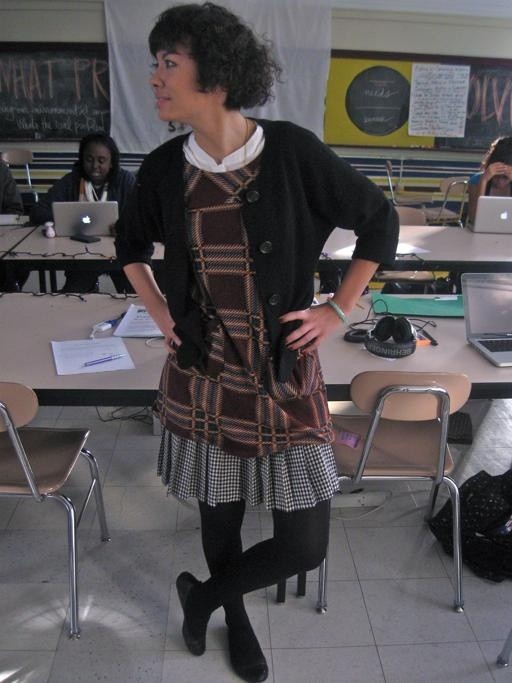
385;160;434;208
1;148;40;206
373;208;434;295
417;176;472;227
317;370;472;615
0;381;110;641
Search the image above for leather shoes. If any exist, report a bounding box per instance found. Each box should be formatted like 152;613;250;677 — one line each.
176;572;207;655
225;612;268;683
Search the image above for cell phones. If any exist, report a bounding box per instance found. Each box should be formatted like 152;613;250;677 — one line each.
70;235;101;243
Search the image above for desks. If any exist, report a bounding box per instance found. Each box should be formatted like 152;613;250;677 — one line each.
0;293;512;400
1;224;512;347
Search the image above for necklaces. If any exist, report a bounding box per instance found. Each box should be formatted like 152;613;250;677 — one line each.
191;121;249;197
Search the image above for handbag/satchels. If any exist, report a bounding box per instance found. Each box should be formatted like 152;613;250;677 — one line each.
427;470;512;583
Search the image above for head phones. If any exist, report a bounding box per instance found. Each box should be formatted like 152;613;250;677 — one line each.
365;316;418;359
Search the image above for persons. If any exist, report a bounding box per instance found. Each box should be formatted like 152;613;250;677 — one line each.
30;133;137;294
114;3;400;683
468;137;512;224
0;159;25;291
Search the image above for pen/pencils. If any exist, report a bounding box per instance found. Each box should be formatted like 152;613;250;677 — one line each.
85;353;126;366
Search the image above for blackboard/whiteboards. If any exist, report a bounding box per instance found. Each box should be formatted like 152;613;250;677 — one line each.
325;50;512;152
0;40;110;143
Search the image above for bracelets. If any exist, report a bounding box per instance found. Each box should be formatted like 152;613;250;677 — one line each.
327;298;349;323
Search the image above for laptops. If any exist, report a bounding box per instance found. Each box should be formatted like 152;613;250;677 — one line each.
461;273;512;368
52;201;118;237
468;196;512;234
